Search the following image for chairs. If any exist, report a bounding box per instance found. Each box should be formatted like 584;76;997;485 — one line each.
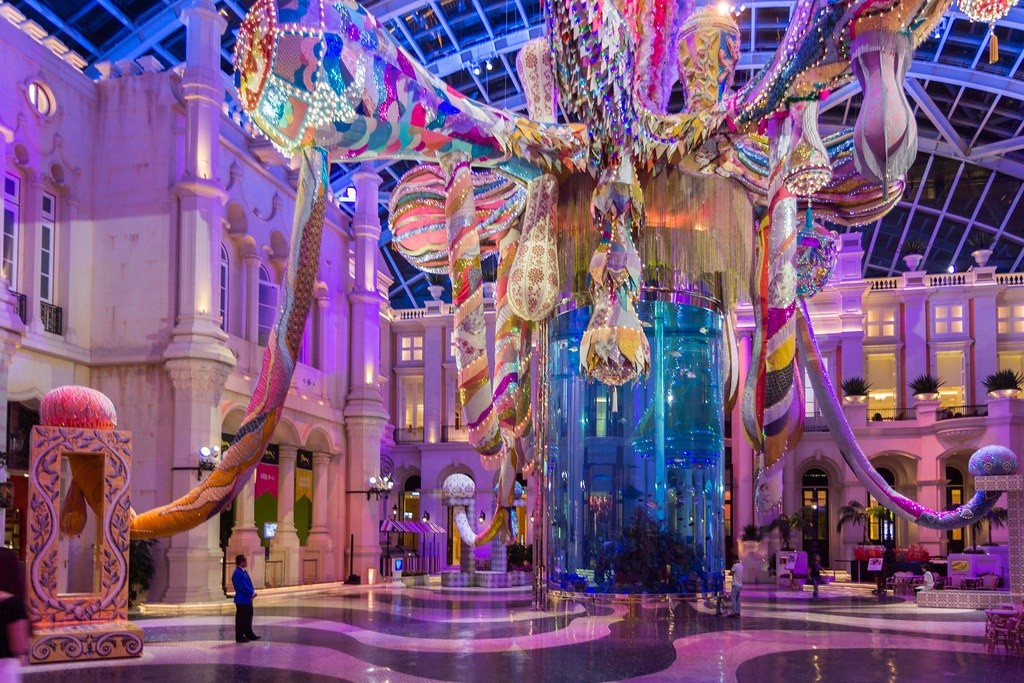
982;601;1024;660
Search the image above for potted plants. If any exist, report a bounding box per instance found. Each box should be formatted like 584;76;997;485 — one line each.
840;377;874;403
129;538;160;607
907;373;946;400
981;367;1024;399
760;507;812;583
894;237;928;271
948;505;1009;587
837;499;891;560
968;230;995;267
742;524;761;552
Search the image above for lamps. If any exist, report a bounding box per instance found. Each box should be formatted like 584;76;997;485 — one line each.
530;511;534;523
200;446;220;471
479;510;485;523
368;473;393;491
423;510;429;523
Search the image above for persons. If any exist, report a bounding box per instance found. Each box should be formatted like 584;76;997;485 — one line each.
913;564;933;604
726;554;743;618
231;554;262;643
808;553;826;602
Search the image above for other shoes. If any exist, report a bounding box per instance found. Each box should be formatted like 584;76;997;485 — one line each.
245;634;260;640
727;613;740;618
236;638;250;642
